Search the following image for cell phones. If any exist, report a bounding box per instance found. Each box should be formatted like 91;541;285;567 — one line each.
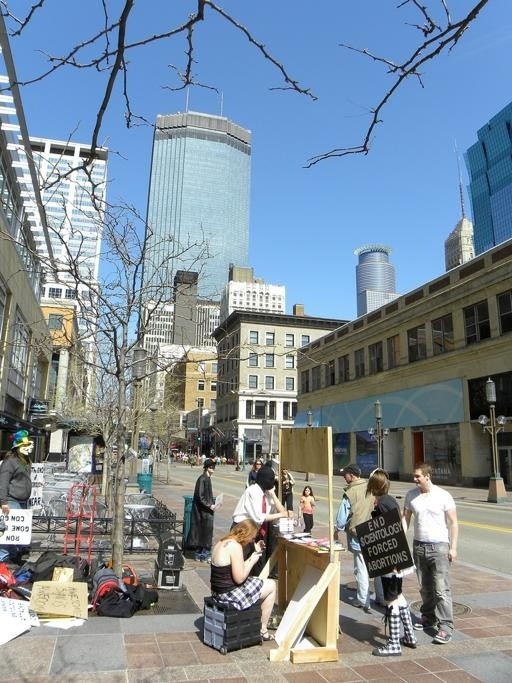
258;550;264;554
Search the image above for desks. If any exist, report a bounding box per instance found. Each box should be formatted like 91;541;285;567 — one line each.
277;534;345;664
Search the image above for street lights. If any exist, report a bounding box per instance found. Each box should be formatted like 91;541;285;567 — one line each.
368;398;390;472
303;410;316;479
149;403;158;462
478;375;508;504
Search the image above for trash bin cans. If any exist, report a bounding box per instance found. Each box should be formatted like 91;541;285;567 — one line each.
181;495;216;551
137;473;153;494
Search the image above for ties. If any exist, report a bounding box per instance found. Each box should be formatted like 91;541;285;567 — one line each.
261;495;267;536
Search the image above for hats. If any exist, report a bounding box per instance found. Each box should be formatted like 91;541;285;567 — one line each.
340;464;361;476
203;459;215;468
10;430;30;450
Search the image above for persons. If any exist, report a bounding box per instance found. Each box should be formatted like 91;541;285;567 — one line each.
0;429;35;563
172;451;234;468
187;459;220;563
230;467;293;577
211;519;276;641
245;461;317;533
334;461;457;657
147;451;153;473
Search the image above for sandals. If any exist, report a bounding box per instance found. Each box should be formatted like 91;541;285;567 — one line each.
260;631;273;640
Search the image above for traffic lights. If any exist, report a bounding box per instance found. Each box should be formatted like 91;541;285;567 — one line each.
198;437;201;445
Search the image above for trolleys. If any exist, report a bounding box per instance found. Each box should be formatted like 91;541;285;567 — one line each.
64;483;96;577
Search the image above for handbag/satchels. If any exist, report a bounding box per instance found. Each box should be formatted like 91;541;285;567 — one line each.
0;547;158;617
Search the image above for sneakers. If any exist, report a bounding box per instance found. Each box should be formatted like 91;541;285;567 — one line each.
433;630;451;644
414;616;437;630
352;599;370;610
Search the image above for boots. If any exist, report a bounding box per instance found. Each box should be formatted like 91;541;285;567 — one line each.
399;607;417;648
372;605;400;656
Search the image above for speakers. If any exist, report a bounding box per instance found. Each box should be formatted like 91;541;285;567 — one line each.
158;539;185;568
